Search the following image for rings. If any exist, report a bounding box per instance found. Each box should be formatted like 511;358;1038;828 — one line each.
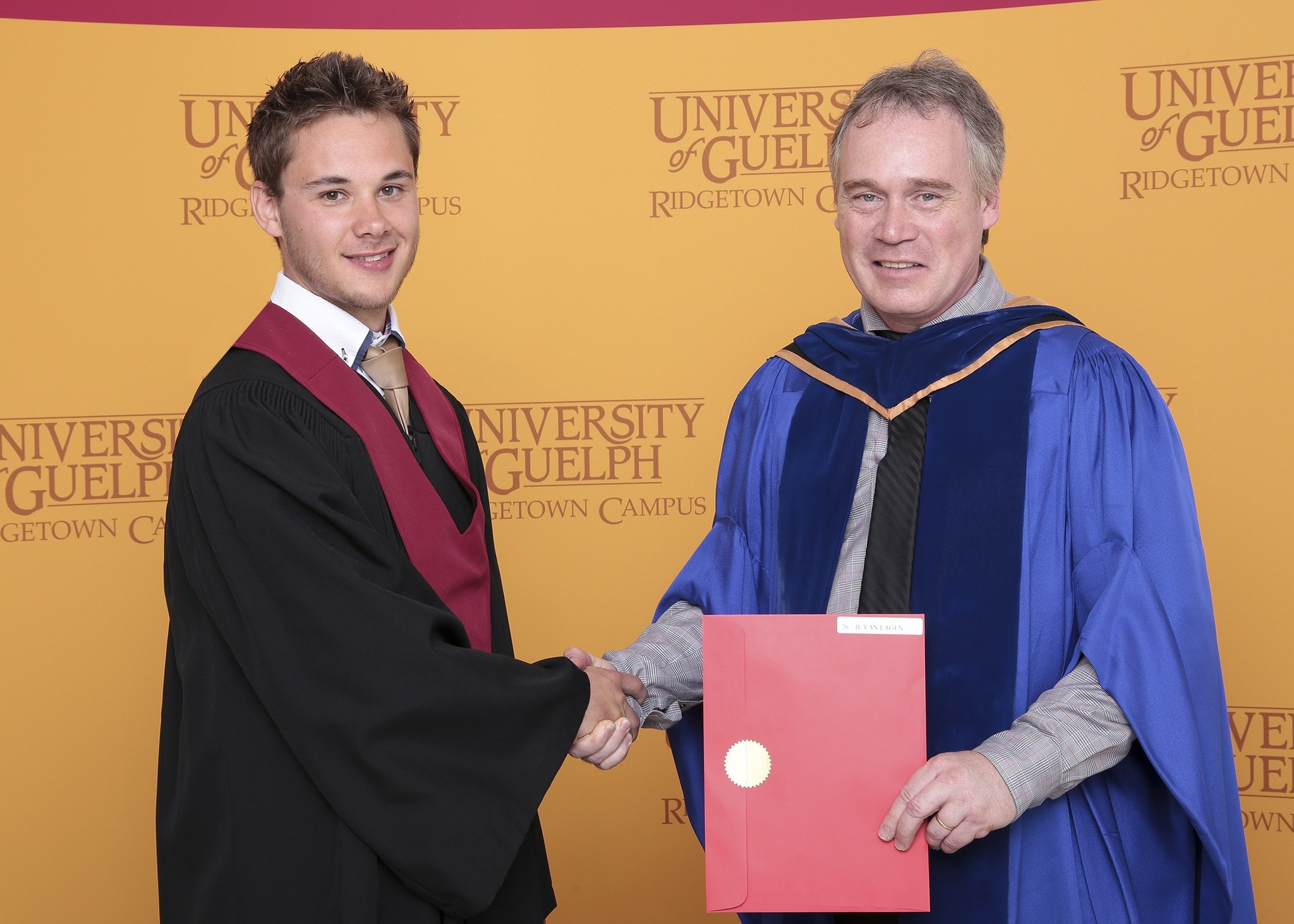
935;811;956;831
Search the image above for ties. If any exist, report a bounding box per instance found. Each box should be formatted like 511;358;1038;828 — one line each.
858;329;929;615
360;335;412;436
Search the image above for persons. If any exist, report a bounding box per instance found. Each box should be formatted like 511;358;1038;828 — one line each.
153;59;644;922
560;53;1258;923
